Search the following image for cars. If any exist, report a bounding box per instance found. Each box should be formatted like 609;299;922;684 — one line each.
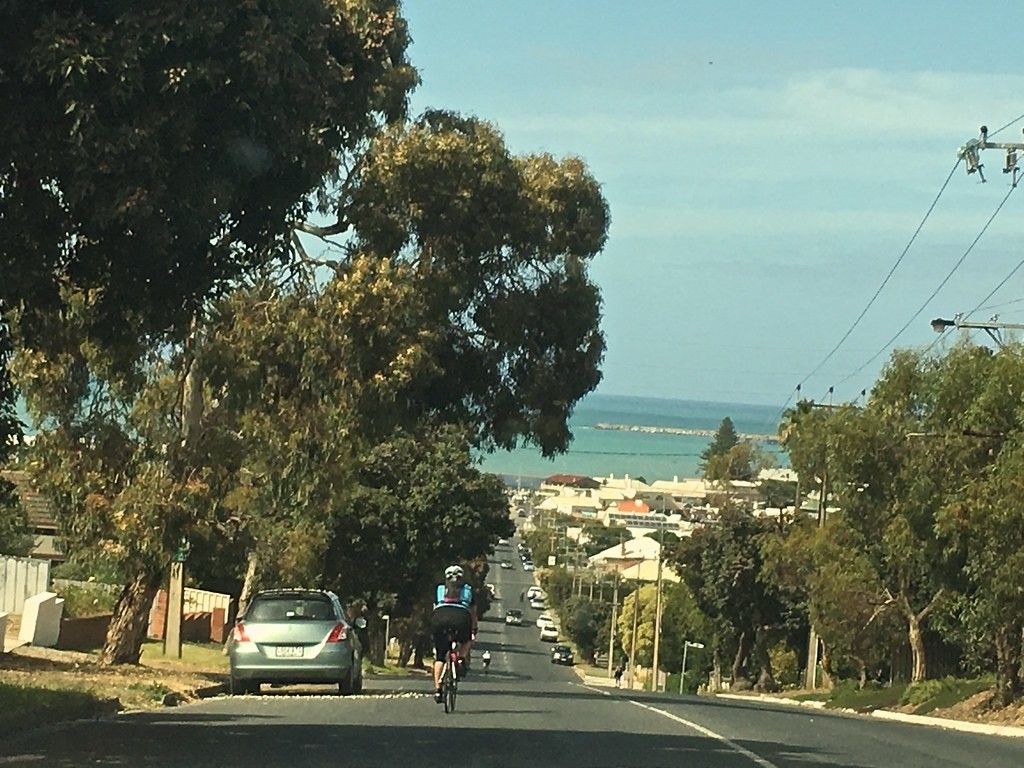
230;588;363;696
500;559;512;569
536;615;558;642
505;609;524;625
527;586;545;609
517;542;534;571
551;645;575;665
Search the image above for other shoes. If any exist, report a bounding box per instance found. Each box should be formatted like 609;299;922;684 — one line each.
457;658;466;679
434;690;442;703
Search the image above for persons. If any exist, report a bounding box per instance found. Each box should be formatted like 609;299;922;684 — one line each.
614;666;623;687
430;565;479;704
482;650;491;668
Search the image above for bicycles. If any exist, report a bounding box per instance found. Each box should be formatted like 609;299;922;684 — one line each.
436;628;466;714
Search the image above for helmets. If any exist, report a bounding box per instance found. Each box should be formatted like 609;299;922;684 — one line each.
444;565;464;579
